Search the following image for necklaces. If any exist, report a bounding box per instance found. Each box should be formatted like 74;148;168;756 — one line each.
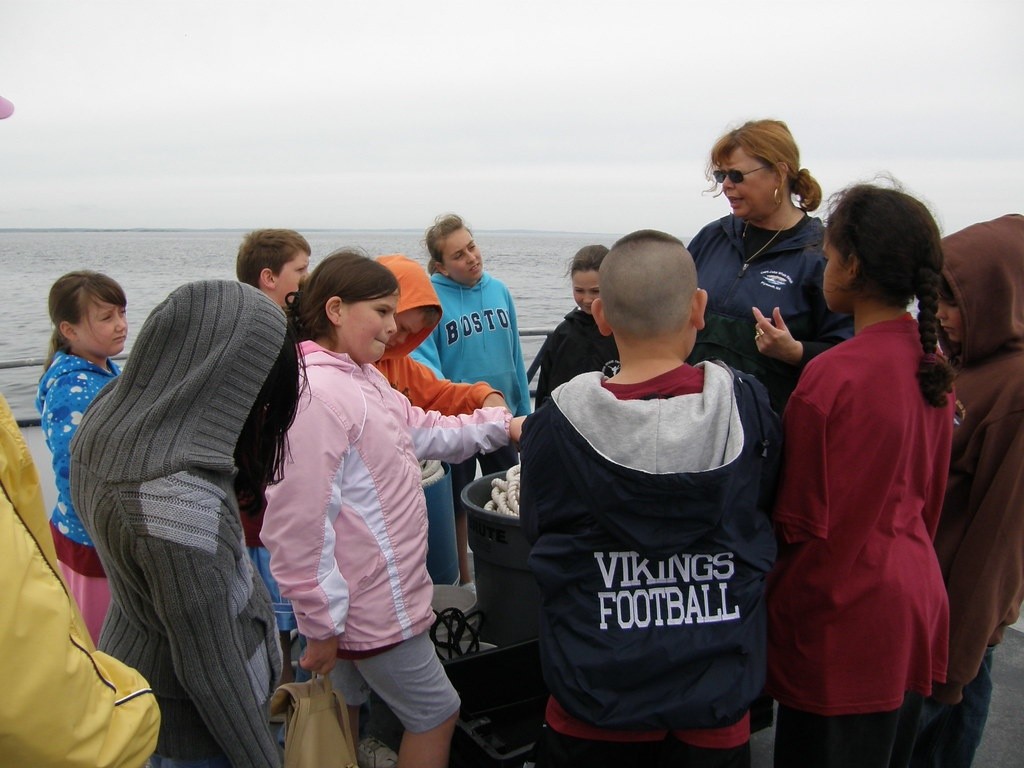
742;217;789;262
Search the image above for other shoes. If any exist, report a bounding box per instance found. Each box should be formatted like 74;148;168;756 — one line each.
461;582;477;596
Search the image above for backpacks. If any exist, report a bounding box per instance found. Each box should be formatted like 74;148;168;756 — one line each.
268;669;358;768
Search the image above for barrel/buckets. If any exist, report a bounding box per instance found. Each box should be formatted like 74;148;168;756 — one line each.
418;460;460;590
462;470;541;646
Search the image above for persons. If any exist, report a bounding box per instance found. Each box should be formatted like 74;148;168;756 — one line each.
0;120;1024;768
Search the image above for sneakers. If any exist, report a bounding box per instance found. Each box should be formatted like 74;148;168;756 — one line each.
356;734;399;768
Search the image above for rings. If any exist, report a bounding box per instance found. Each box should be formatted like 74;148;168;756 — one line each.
754;331;760;340
758;328;765;336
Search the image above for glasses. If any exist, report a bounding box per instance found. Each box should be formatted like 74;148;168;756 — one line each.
712;164;773;184
